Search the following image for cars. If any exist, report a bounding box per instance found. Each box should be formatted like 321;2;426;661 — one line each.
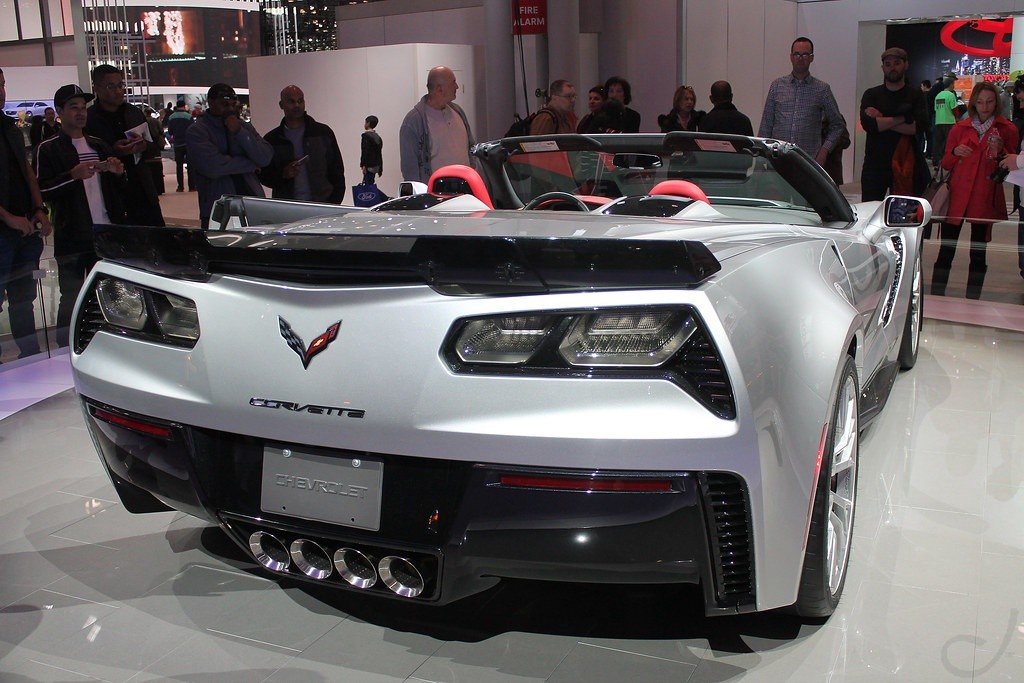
4;100;55;121
130;102;160;119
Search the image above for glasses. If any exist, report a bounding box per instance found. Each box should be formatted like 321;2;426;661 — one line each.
97;82;126;91
681;86;692;89
214;94;238;102
792;53;813;59
554;95;577;101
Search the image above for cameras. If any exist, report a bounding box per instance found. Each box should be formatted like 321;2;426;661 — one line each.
991;154;1010;183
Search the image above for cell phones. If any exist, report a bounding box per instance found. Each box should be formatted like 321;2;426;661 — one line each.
295;155;309;166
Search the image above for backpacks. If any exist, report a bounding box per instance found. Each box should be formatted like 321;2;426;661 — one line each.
504;109;559;138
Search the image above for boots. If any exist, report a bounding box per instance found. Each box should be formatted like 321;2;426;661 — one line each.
966;263;987;300
931;263;951;296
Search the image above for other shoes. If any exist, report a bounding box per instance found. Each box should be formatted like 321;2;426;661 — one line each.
176;188;184;192
934;166;939;171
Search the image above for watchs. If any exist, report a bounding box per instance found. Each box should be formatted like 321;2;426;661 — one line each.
37;207;48;215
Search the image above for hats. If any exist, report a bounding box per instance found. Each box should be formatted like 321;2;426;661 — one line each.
54;84;95;108
944;72;958;79
1004;81;1015;88
881;47;907;61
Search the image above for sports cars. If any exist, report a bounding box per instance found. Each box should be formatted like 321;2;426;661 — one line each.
68;129;935;620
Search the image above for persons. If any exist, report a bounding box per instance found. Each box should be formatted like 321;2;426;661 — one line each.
860;48;928;203
399;66;477;186
30;107;62;175
576;77;640;180
360;115;383;186
992;74;1024;279
757;37;846;205
529;80;578;200
0;68;53;359
930;80;1019;301
920;71;967;187
142;101;205;193
820;110;851;189
36;85;129;351
255;85;345;206
657;86;706;178
184;83;274;230
695;80;754;153
83;64;166;226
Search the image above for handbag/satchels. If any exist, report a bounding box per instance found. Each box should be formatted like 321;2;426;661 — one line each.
352;173;381;207
921;162;955;223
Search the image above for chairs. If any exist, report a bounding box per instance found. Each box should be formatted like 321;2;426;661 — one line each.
649;179;710;206
427;165;495;211
